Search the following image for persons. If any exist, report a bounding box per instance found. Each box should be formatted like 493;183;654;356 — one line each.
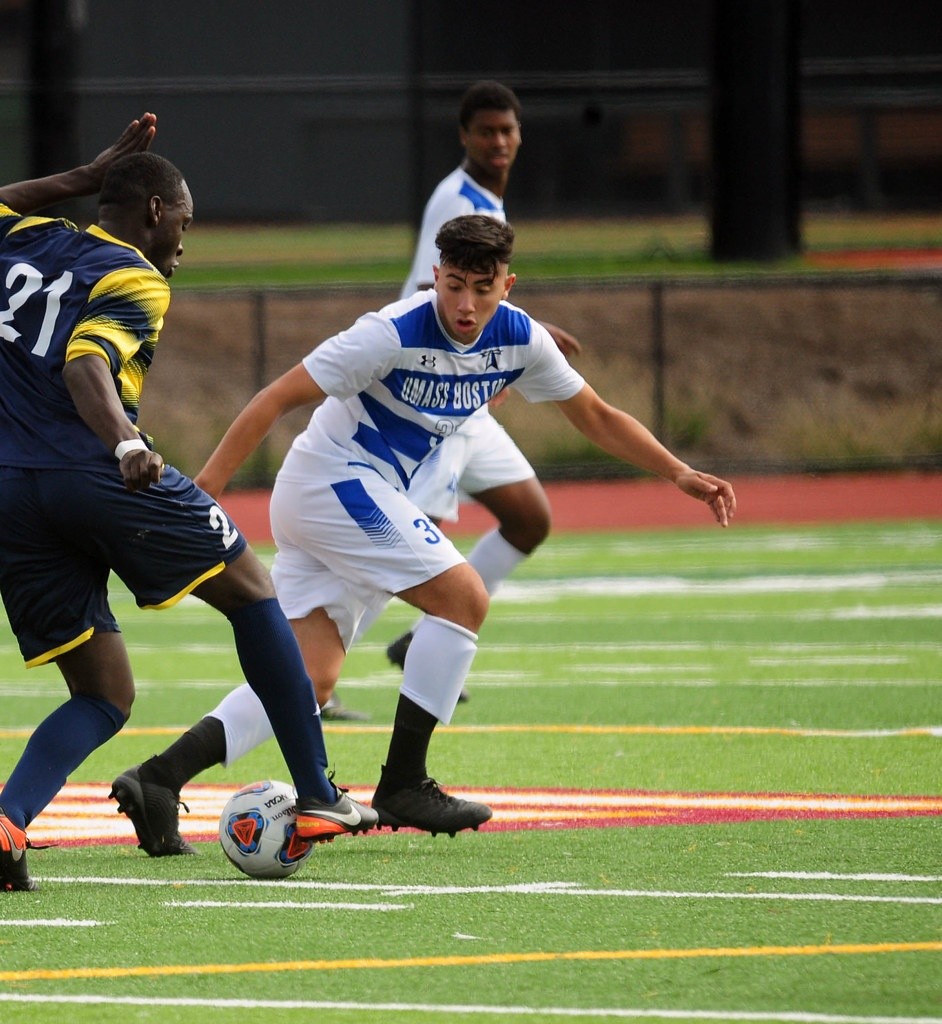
0;78;741;894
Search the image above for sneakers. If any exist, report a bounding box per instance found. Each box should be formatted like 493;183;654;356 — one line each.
110;756;200;856
372;776;493;839
388;634;467;702
321;700;365;721
296;766;379;845
0;808;59;892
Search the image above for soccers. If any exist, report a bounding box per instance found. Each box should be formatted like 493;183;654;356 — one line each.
218;780;316;879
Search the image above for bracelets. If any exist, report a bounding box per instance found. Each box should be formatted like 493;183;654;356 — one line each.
114;439;148;460
